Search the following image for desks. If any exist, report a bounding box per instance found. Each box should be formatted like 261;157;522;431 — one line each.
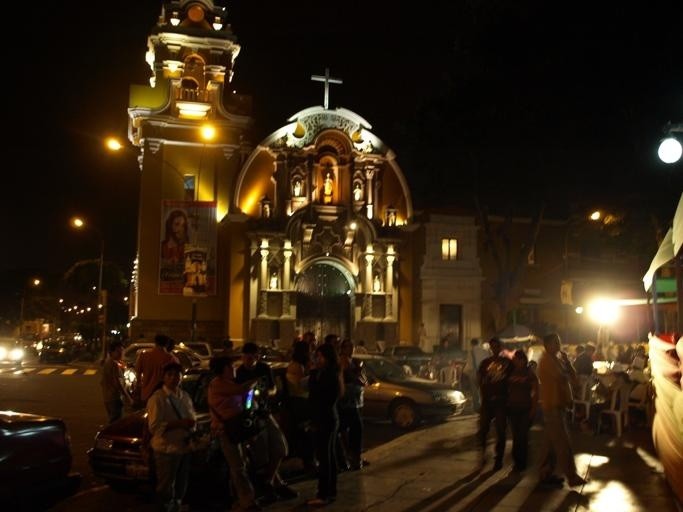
590;361;630;386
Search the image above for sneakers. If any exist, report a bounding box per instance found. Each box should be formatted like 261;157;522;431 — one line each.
540;474;564;484
493;460;502;471
273;483;300;499
304;497;330;505
569;476;590;487
258;482;275;505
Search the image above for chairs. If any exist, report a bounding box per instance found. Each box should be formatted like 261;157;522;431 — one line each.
402;362;460;386
563;372;591;422
596;382;629;436
628;375;653;427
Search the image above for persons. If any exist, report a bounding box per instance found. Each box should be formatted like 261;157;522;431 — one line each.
429;333;652;485
100;330;369;512
160;212;187;290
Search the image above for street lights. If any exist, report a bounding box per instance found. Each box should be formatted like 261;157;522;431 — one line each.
72;214;105;295
191;122;217;339
17;276;99;328
99;134;197;341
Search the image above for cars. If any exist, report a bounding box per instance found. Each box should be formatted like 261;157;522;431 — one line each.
378;344;433;373
0;329;91;365
350;352;467;431
98;339;213;419
86;361;292;492
0;410;72;500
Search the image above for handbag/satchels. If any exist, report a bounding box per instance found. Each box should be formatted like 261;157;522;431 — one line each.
223;410;260;446
162;424;200;456
